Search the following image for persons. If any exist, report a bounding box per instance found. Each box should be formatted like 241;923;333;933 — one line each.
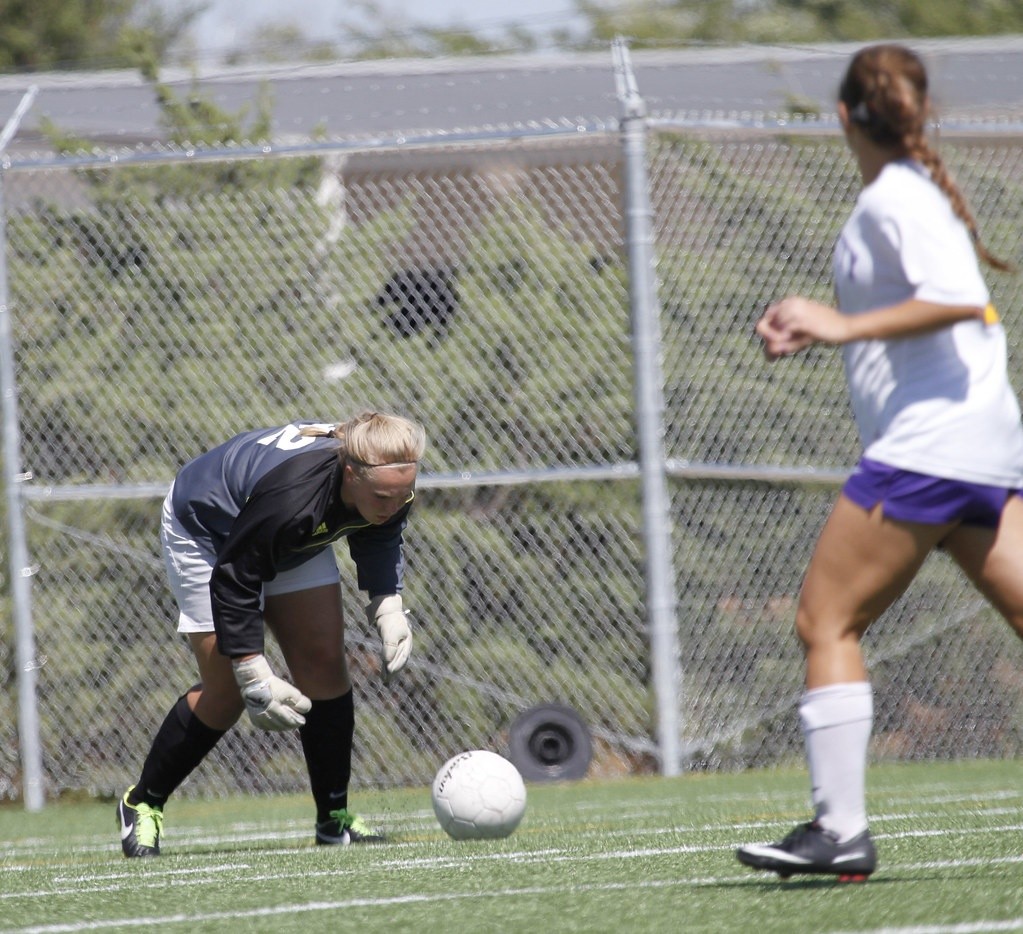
735;45;1023;882
114;411;427;858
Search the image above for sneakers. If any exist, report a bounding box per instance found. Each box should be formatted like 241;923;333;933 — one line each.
315;809;384;846
737;823;876;884
116;785;163;859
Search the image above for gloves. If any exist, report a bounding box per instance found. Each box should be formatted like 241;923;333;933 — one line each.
366;593;412;682
232;656;311;731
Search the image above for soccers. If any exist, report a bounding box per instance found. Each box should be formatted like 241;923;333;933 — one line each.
431;748;526;840
